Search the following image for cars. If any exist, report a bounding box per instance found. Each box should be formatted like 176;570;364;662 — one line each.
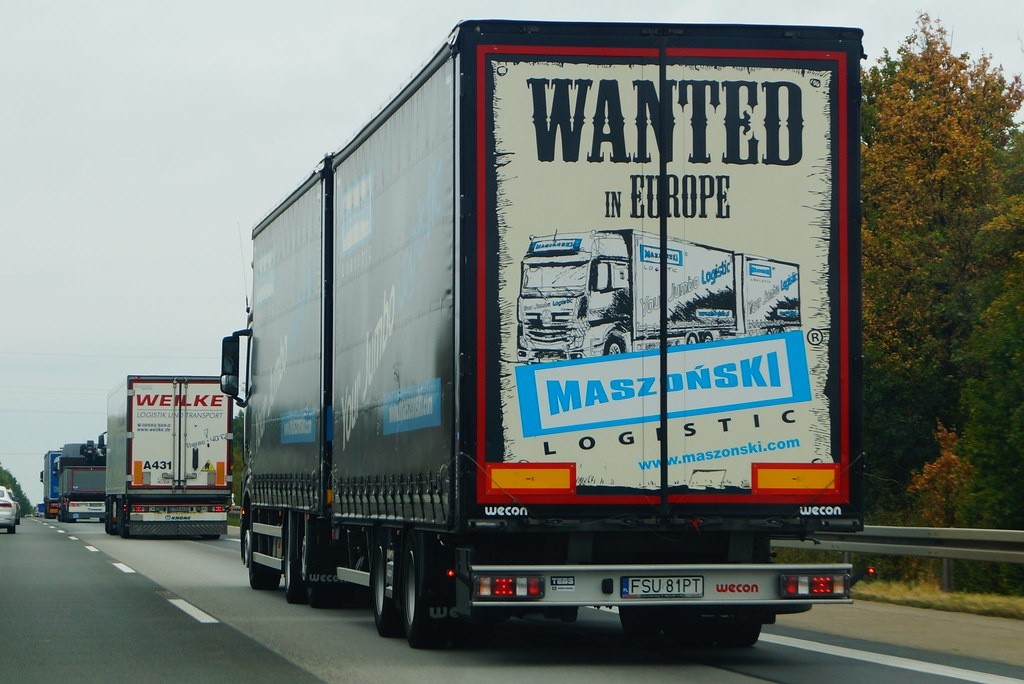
0;485;20;535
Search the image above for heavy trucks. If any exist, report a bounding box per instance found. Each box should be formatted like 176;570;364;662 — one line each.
34;439;106;523
97;374;234;539
219;16;873;651
